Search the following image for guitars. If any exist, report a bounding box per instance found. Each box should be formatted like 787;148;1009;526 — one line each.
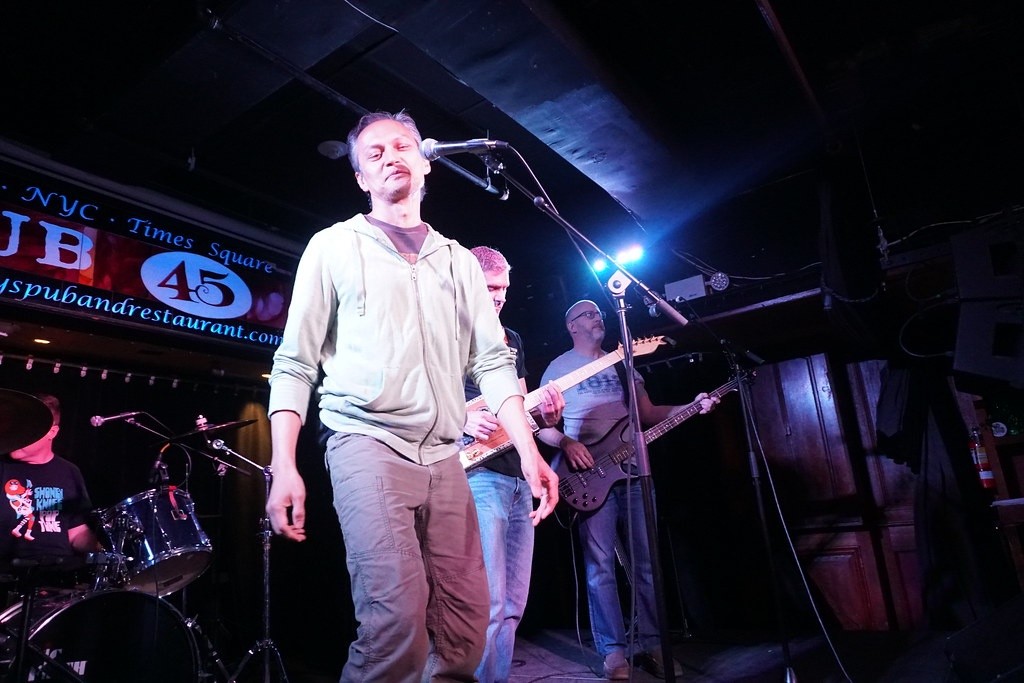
456;334;667;471
545;370;747;511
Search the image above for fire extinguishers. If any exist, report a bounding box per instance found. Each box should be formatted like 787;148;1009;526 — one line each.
967;427;995;489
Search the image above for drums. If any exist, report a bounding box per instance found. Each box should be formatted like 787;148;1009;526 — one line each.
97;484;216;596
12;586;203;683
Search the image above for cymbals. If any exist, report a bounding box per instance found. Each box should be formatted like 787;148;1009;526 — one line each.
0;387;56;456
158;419;258;438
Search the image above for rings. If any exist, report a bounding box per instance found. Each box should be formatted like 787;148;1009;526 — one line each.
546;402;553;406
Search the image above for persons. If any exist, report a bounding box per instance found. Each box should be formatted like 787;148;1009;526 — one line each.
265;113;558;683
458;246;567;681
0;393;104;570
530;299;720;682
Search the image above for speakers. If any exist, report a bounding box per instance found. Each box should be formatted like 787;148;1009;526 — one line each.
950;203;1024;299
943;590;1024;683
954;300;1024;389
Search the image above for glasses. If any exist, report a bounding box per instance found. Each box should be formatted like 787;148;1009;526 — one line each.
570;311;607;322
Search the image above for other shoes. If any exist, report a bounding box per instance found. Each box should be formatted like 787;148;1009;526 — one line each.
646;648;683;678
603;653;630;679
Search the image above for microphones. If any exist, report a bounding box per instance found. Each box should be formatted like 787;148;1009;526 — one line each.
151;444;170;476
419;137;509;161
649;296;684;317
90;409;140;427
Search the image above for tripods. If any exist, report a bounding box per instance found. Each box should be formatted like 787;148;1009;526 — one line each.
212;437;290;683
648;444;747;649
